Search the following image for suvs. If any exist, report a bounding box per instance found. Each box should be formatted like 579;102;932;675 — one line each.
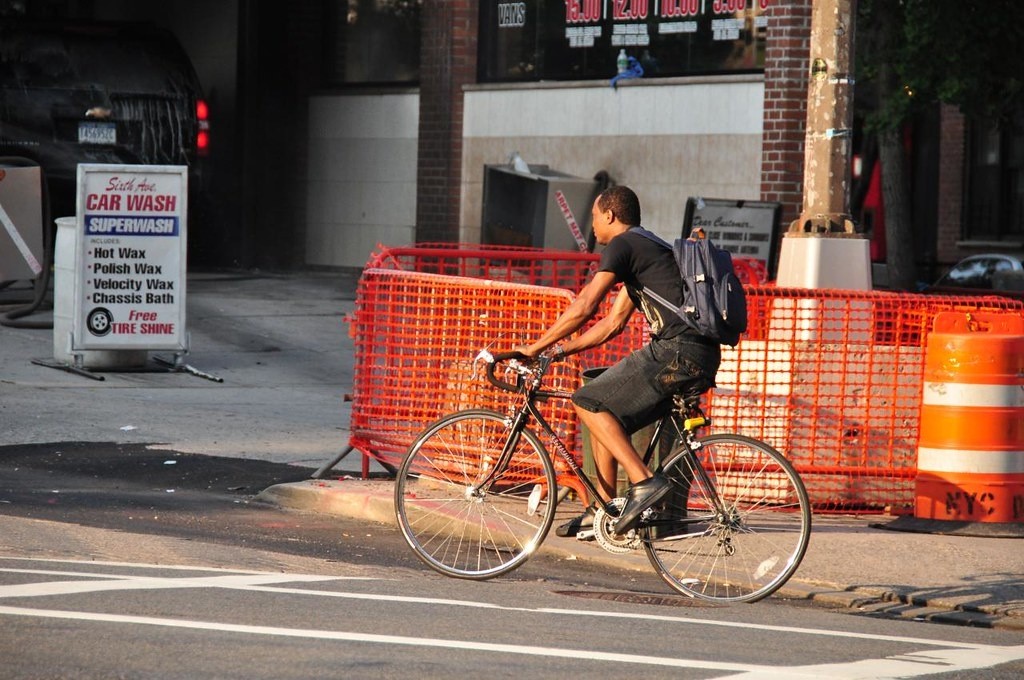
0;14;210;228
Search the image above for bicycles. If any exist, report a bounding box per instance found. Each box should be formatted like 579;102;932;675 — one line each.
394;340;812;605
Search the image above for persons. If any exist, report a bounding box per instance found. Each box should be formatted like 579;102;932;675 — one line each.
513;185;722;538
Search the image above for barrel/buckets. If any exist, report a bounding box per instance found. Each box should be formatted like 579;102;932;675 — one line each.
581;368;693;540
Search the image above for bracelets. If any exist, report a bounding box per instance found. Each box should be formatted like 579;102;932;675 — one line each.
557;346;564;362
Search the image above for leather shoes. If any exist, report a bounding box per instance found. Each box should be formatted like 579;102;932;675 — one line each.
556;501;611;537
616;473;673;535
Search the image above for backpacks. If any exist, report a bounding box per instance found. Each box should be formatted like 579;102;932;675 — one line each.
627;227;747;347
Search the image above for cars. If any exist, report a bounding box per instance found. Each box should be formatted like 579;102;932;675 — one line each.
917;249;1024;309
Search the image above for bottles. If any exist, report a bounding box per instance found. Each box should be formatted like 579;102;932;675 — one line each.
617;50;627;72
511;151;531;174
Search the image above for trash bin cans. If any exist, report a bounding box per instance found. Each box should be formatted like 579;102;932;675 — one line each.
479;161;615;289
53;215;148;367
